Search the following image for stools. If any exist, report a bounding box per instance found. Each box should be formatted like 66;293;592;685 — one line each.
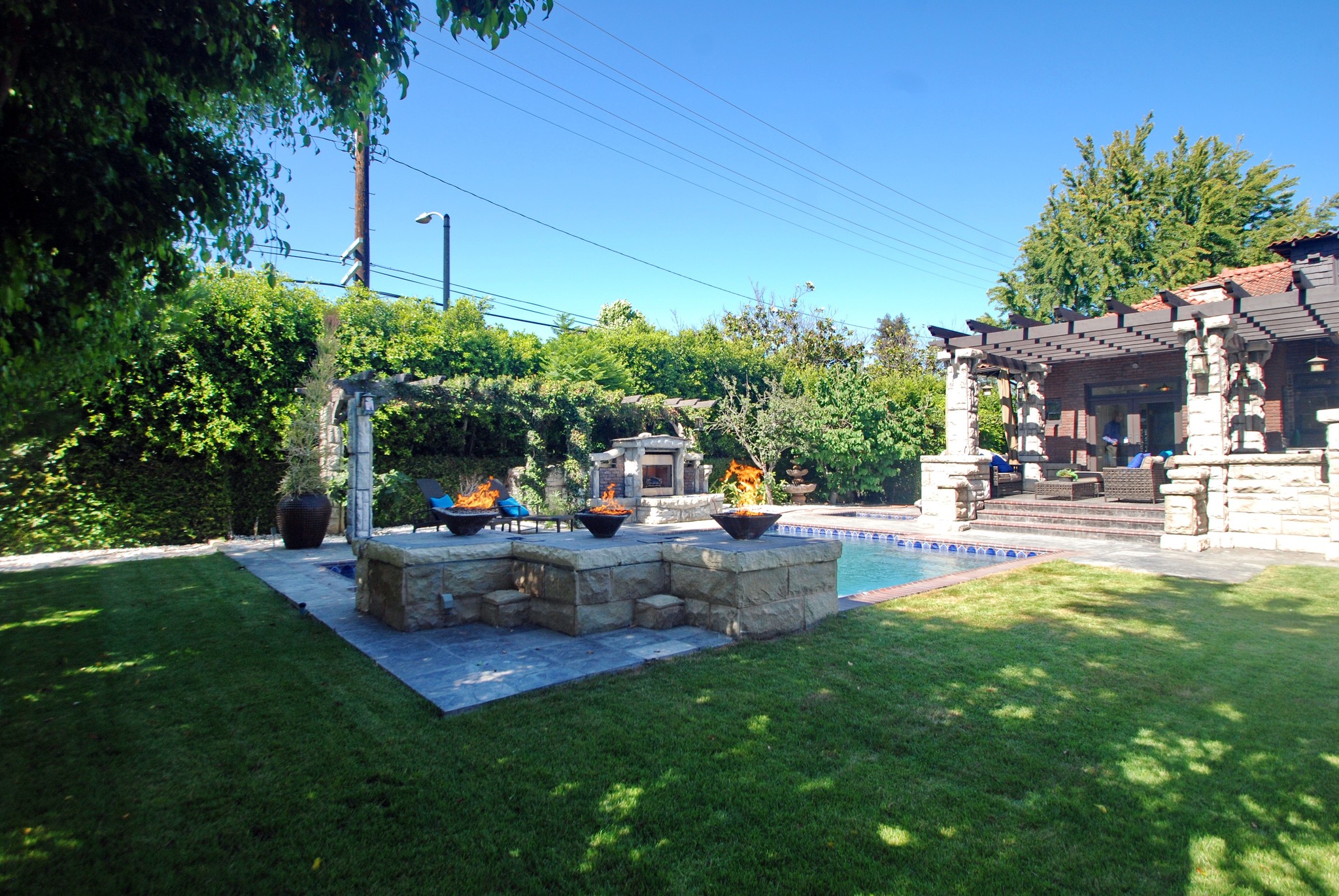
1071;471;1104;497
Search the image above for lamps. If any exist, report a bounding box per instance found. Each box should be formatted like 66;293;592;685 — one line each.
1306;342;1329;373
1156;382;1173;392
1139;383;1149;390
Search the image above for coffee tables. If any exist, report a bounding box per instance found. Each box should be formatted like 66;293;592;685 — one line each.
1034;478;1097;501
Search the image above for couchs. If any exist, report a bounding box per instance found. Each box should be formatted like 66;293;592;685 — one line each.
1164;454;1179;484
1103;456;1166;503
990;463;1023;497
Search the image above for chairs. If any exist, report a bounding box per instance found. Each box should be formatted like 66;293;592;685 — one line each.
410;479;512;533
483;478;575;534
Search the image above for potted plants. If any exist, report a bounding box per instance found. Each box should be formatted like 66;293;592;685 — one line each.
1056;468;1079;483
273;308;347;549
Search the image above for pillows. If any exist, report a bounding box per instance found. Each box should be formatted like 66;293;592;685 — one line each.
497;496;529;516
979;453;992;467
990;455;1014;473
1159;449;1173;461
429;494;454;508
1126;454;1151;468
